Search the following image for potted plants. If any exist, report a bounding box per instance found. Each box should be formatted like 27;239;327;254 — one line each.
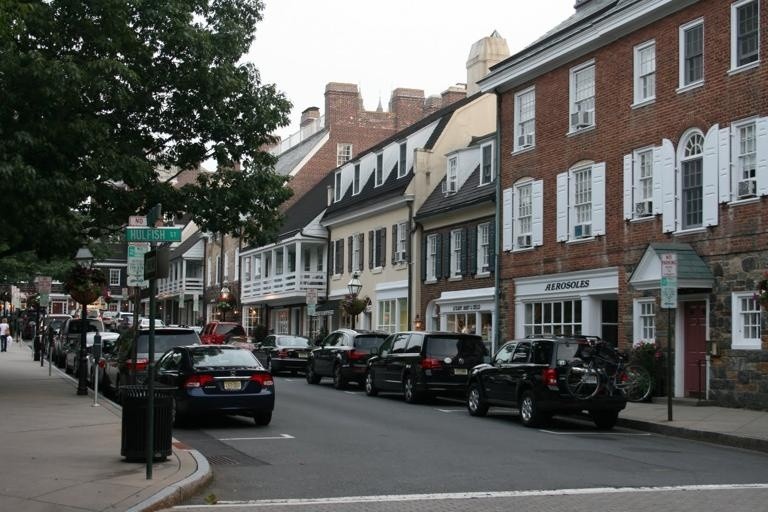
216;301;233;313
25;292;48;311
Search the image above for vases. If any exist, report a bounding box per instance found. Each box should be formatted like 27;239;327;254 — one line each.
343;303;367;316
69;285;99;305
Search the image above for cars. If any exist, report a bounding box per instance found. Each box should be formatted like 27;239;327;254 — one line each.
252;334;315;375
8;309;120;391
137;344;275;427
223;335;261;346
114;311;202;333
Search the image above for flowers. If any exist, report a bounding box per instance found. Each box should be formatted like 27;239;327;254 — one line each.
340;293;373;315
52;265;114;305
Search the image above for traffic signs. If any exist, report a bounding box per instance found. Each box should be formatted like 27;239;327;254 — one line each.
126;226;182;242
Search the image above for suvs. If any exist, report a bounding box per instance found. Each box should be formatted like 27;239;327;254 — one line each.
467;333;627;430
199;321;247;345
363;330;486;403
102;327;202;405
305;327;391;389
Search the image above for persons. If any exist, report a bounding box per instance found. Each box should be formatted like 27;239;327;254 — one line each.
0;318;10;352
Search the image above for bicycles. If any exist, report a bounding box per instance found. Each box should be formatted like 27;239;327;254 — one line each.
564;335;651;402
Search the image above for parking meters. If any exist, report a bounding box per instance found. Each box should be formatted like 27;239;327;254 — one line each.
89;329;102;408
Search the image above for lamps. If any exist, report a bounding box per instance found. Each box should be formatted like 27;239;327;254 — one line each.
415;312;425;331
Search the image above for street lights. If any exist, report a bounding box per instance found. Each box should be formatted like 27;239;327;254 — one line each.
220;277;230;321
348;271;362;329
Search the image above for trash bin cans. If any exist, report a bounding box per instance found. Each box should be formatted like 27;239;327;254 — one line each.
118;385;178;461
